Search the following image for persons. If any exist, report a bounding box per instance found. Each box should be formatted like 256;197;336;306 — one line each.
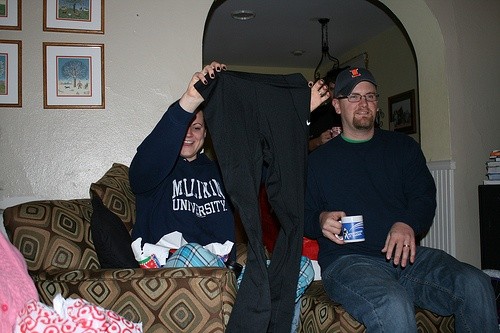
128;62;330;333
303;67;500;333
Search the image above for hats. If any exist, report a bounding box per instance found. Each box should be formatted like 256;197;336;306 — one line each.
334;68;378;96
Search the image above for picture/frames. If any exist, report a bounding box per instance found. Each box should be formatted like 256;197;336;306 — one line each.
0;39;22;108
43;0;105;34
43;42;105;109
0;0;22;30
388;89;417;134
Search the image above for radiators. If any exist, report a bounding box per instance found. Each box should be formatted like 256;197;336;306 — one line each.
420;160;456;259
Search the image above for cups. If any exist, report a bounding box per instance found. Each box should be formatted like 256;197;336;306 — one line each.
334;215;365;243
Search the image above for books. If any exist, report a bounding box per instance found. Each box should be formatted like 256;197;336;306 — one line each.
484;151;500;185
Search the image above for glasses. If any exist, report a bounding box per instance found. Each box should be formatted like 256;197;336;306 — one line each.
337;94;379;103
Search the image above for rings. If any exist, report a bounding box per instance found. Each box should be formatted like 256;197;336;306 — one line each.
403;244;409;248
318;91;323;96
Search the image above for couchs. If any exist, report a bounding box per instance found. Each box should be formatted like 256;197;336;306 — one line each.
3;197;457;333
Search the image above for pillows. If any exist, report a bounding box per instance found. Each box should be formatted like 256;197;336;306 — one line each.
90;163;139;268
